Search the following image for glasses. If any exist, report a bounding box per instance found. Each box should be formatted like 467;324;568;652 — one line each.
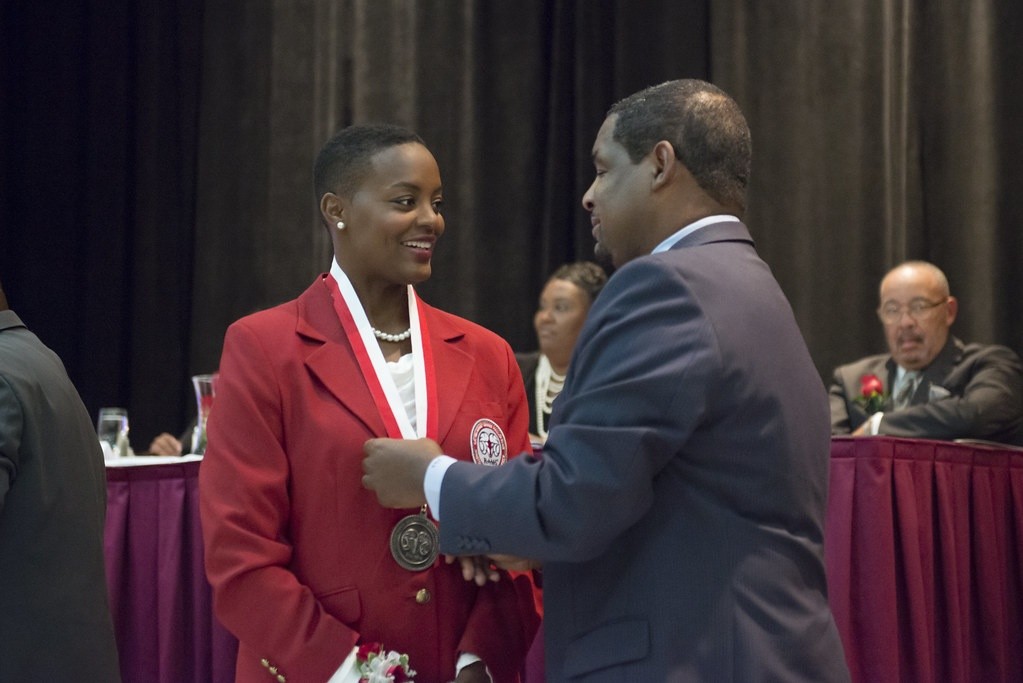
876;296;950;324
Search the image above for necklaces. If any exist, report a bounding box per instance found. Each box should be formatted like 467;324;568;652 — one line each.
368;326;414;343
543;370;569;403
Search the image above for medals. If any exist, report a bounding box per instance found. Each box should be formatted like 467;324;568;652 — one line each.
390;514;440;571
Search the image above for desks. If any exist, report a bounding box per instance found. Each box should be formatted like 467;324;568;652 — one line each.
101;435;1023;682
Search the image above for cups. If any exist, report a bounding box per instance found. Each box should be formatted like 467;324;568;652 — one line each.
193;368;220;456
97;408;129;457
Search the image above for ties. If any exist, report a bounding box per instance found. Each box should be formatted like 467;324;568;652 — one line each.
895;368;922;407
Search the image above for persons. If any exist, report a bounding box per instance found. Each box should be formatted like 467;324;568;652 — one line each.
828;261;1022;445
512;261;610;441
0;290;127;682
364;73;853;683
198;120;544;683
142;411;199;456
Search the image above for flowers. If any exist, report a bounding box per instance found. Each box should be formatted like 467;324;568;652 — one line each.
353;640;418;682
201;394;213;443
853;371;887;416
